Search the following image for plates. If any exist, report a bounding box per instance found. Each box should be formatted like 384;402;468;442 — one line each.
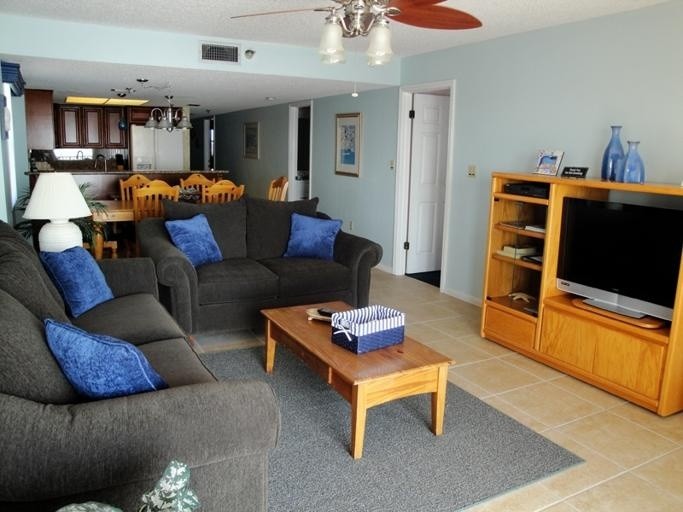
304;307;334;321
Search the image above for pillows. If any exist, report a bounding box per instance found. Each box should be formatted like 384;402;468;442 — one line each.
43;319;165;398
284;212;343;261
166;214;222;270
39;247;115;315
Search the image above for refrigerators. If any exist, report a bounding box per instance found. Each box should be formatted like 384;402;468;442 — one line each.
129;124;183;172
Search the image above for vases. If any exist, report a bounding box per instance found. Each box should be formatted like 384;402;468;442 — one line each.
601;125;627;183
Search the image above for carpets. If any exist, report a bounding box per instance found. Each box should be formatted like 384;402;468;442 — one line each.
196;344;585;512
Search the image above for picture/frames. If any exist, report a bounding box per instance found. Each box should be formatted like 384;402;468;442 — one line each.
534;149;565;176
144;95;193;131
242;121;260;161
334;113;361;177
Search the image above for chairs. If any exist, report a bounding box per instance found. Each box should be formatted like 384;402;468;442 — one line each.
91;172;289;258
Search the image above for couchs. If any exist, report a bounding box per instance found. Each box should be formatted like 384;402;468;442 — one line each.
137;211;383;334
0;219;281;512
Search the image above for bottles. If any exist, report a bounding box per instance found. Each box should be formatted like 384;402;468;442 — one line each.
602;124;624;180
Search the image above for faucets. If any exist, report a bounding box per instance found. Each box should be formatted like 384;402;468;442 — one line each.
76;151;84;161
94;153;107;172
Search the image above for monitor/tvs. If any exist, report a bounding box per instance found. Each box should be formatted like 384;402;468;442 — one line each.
556;197;683;322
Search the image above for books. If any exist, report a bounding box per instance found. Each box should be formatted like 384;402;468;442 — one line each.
501;218;544;230
525;223;546;234
523;304;539;314
503;242;536;254
495;250;537;259
520;254;543;265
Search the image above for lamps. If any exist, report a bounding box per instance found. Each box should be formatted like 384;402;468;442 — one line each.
314;13;393;68
22;172;93;252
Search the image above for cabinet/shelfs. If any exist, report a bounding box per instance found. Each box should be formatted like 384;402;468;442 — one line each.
58;106;126;149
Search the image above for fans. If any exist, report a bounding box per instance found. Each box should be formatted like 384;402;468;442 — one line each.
229;0;482;30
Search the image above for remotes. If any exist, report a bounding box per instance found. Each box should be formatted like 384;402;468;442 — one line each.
318;308;337;317
